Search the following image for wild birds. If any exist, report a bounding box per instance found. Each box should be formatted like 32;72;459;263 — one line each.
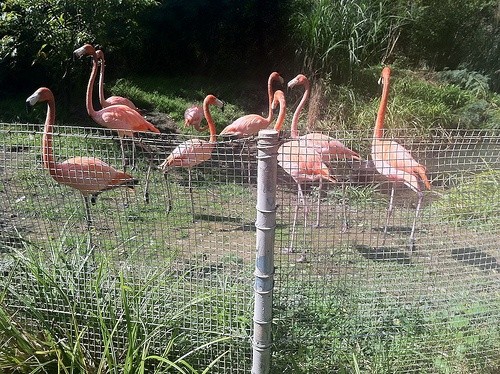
91;50;141;170
371;66;430;267
73;44;160;207
159;94;225;224
26;88;139;236
285;74;363;233
271;90;339;263
183;106;216;136
217;72;284;191
